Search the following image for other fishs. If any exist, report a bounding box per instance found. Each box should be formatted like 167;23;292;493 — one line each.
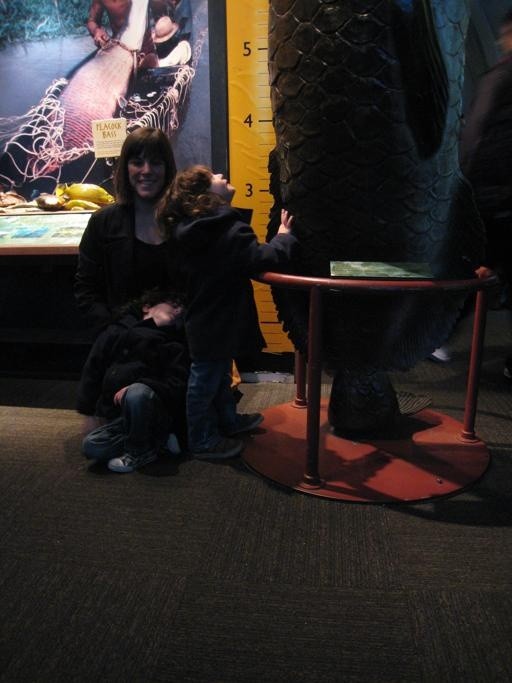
265;0;487;377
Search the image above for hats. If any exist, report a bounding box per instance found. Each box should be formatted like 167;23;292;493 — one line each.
150;15;179;42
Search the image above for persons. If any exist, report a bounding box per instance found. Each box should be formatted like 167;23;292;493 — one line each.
155;161;298;461
86;0;192;68
70;126;192;477
456;8;511;379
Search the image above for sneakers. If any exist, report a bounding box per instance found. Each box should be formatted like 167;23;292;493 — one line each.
225;412;265;434
193;437;244;459
108;449;158;474
163;435;180;452
